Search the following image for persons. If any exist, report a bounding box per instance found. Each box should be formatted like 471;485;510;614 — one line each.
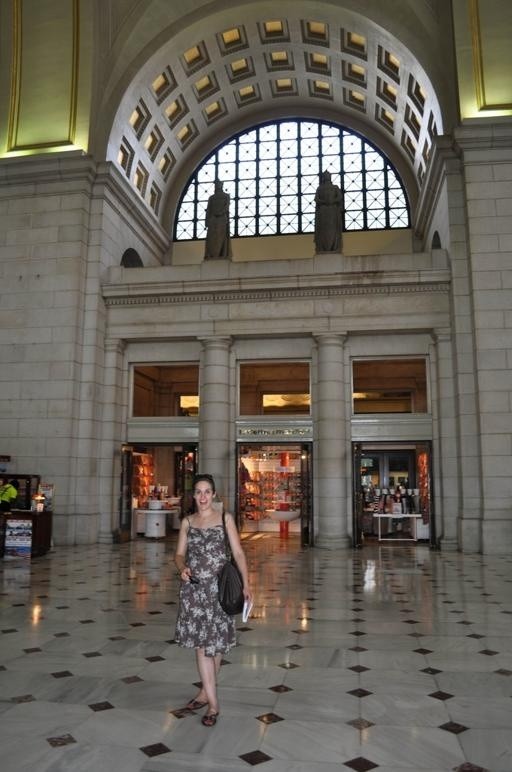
203;180;234;261
0;479;20;512
0;479;5;486
174;473;254;727
313;169;344;253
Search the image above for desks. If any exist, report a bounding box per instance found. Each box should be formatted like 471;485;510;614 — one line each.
134;508;178;539
373;512;422;542
265;509;300;539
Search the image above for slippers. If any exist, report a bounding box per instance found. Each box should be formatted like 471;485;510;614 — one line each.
202;713;218;726
186;699;209;709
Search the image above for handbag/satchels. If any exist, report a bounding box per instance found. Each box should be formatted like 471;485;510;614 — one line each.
218;561;244;615
